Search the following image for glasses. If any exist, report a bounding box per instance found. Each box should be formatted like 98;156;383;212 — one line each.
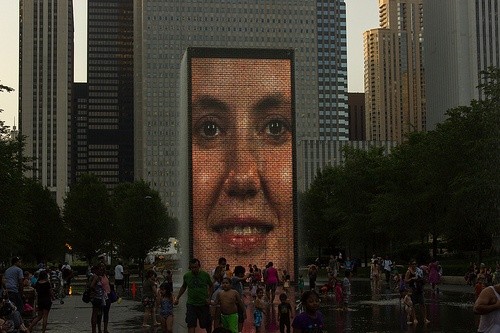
15;257;21;261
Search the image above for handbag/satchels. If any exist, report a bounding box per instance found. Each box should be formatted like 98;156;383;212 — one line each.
23;303;33;312
109;284;119;302
82;289;91;303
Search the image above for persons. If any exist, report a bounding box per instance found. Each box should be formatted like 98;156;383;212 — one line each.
308;266;317;291
399;259;442;325
0;256;72;333
86;261;120;333
344;256;354;277
292;290;324;333
321;274;351;304
114;261;129;297
471;261;500;302
166;270;173;287
175;259;214;333
191;59;294;283
159;285;176;333
473;284;500;333
329;256;338;277
211;257;293;333
142;270;160;327
371;255;393;292
298;274;305;294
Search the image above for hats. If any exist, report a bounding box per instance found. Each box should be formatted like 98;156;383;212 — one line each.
478;274;485;279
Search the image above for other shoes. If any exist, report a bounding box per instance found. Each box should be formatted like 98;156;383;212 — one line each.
143;324;150;327
424;319;431;327
153;323;160;327
407;322;412;325
414;320;420;327
